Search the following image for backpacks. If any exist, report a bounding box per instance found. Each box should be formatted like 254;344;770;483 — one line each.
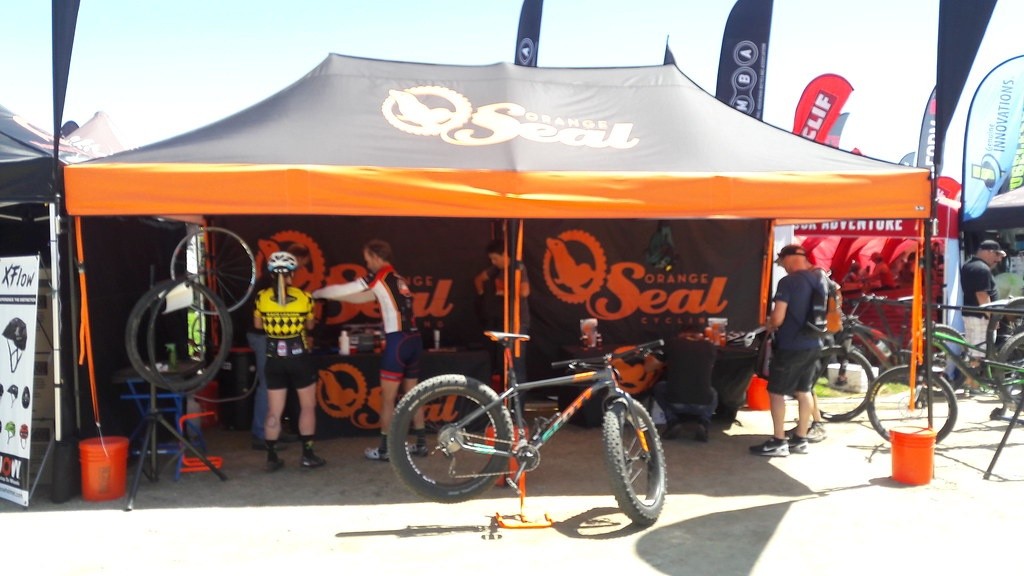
788;271;828;336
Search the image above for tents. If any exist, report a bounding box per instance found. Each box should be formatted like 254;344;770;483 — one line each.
0;107;128;503
63;52;935;432
793;184;1024;282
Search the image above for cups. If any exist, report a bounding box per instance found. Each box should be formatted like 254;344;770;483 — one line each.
165;342;176;365
579;318;598;348
707;318;728;348
77;435;130;501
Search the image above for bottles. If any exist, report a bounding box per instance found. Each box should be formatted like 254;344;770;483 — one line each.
873;338;892;358
338;329;350;357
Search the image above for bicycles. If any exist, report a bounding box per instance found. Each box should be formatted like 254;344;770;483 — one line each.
387;323;669;526
807;293;1024;444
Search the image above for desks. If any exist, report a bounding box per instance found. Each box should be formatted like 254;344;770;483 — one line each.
557;343;758;426
285;348;498;441
110;359;203;482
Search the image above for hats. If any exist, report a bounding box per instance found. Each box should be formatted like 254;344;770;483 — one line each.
979;240;1007;258
775;245;810;264
868;252;883;260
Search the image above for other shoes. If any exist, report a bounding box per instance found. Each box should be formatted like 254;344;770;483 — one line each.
662;420;680;439
694;419;708;443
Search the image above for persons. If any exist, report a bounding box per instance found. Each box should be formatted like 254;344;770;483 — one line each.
806;263;834;443
748;245;828;456
474;239;530;419
654;319;717;438
254;251;325;470
330;240;429;461
840;239;1008;398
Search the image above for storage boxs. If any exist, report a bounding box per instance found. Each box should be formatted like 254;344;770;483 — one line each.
827;363;879;394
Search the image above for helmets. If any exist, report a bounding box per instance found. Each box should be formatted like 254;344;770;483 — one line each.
266;251;300;274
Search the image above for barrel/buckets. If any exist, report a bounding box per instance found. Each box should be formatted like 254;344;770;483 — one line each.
889;425;937;486
195;380;220;430
746;374;772;410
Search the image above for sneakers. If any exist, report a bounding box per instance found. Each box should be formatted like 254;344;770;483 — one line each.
749;438;790;457
365;446;390;462
267;459;284;472
302;453;326;469
789;437;809;454
407;444;428;456
807;422;827;442
